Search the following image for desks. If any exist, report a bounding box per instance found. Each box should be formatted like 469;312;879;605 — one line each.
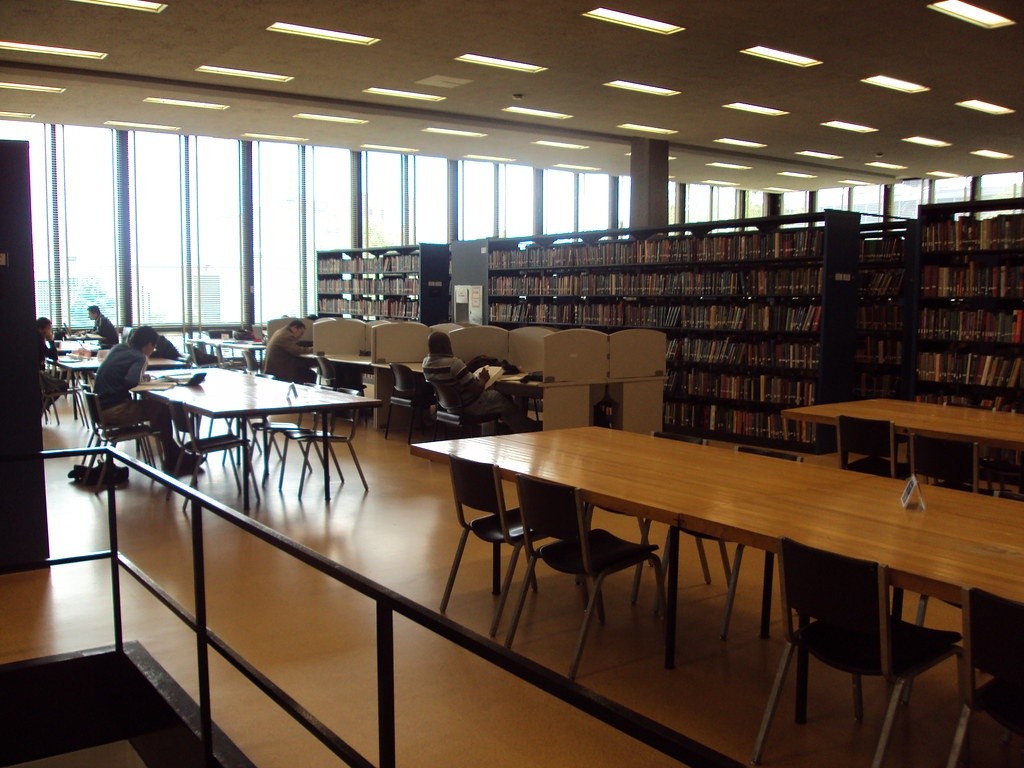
298;351;671;432
410;427;1023;667
780;397;1022;496
46;355;185;419
47;338;112;356
189;334;266;364
130;367;381;510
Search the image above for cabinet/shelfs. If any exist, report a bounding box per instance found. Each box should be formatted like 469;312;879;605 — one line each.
316;243;449;320
913;196;1024;492
845;219;921;444
484;208;863;454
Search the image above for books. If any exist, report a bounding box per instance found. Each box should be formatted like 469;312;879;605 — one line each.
131;380;176;391
488;231;824;444
915;213;1024;465
473;365;504;390
318;255;419;322
854;236;905;399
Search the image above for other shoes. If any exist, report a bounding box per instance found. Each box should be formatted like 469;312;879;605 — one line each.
164;453;205;472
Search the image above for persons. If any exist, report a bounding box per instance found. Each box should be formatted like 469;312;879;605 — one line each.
35;317;66;408
422;332;537;433
262;320;317;384
151;335;182;361
75;306;119;392
93;325;205;466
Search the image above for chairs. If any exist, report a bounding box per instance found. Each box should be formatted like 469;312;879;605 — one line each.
41;332;370;514
386;364;1024;768
